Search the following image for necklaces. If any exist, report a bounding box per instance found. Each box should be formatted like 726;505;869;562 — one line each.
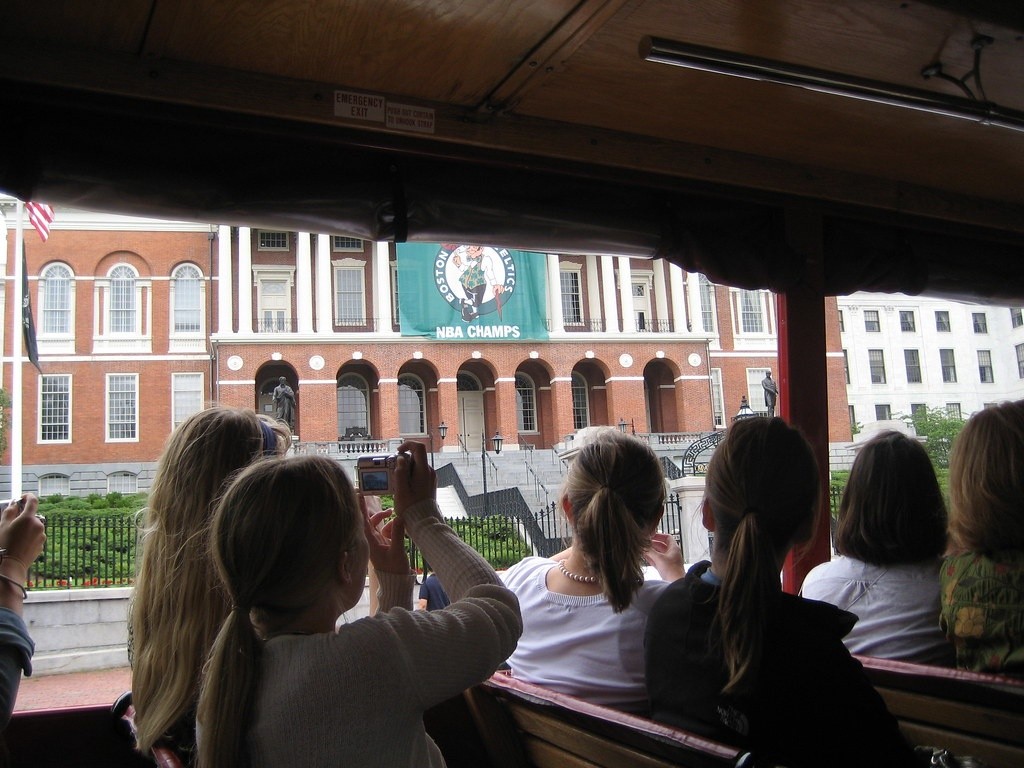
558;558;604;584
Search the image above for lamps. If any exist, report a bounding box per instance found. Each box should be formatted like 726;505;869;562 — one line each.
619;421;629;432
437;422;448;438
493;431;502;453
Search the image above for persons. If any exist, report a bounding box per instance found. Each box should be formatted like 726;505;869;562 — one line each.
801;431;950;664
192;434;525;768
418;562;451;612
495;432;687;709
0;493;47;735
646;413;921;768
938;401;1024;678
272;376;296;425
129;401;296;768
762;371;778;418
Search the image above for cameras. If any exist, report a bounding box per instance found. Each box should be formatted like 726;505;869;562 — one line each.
0;499;46;525
354;451;413;496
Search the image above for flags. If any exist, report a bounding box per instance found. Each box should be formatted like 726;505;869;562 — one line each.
26;201;55;243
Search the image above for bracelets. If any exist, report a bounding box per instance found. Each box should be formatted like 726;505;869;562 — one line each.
0;573;28;599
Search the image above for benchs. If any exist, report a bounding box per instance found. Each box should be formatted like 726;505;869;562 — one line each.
484;670;751;768
852;651;1024;768
111;690;184;768
